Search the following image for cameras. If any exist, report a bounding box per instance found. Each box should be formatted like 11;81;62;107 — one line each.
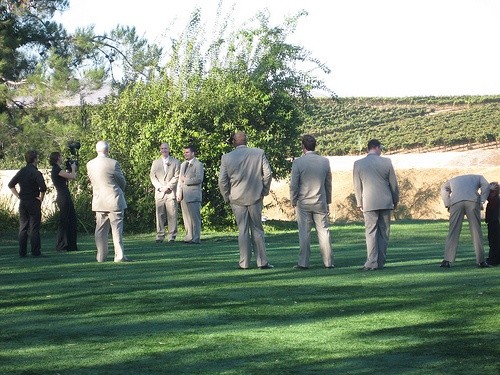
65;158;78;169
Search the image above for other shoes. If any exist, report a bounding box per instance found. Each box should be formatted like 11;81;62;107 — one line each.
327;265;335;268
360;266;376;271
439;261;450;267
293;265;308;269
478;262;491;268
258;263;274;269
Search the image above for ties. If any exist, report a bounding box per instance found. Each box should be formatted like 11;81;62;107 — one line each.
184;162;189;174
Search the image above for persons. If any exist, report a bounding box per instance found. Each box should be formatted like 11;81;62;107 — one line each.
86;140;128;262
176;146;204;244
289;135;335;268
48;152;78;251
485;183;500;265
218;133;272;268
150;143;180;244
353;139;399;270
440;174;489;268
8;150;46;257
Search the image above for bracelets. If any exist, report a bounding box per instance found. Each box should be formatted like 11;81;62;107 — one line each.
18;196;20;198
